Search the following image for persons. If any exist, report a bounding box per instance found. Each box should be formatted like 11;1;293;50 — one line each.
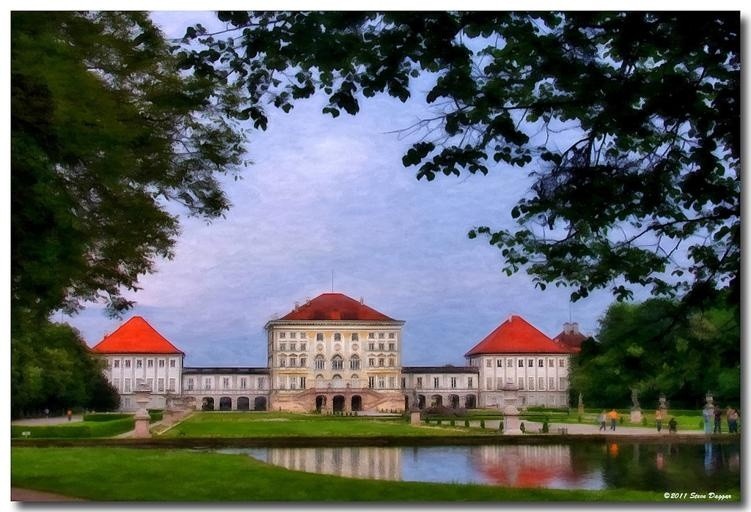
66;408;72;420
608;409;617;431
619;411;625;424
599;409;607;431
657;447;664;470
703;441;740;477
656;408;663;432
668;418;678;432
600;438;608;457
45;408;49;419
703;406;738;434
610;439;618;459
